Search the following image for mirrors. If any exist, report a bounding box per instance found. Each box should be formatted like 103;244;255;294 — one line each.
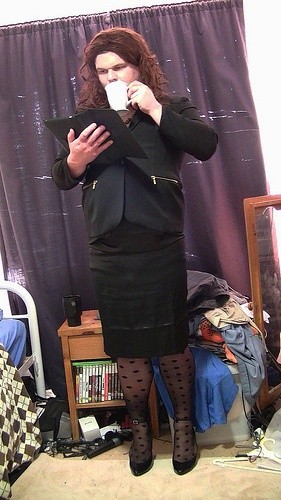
243;196;281;416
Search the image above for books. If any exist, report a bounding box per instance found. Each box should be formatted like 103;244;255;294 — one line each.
76;363;124;404
43;108;149;165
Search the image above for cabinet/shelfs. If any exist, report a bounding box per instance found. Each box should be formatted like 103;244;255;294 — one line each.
57;310;161;444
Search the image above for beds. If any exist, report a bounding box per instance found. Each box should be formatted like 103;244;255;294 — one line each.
0;282;54;499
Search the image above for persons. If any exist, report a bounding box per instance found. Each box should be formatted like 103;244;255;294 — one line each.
51;28;219;477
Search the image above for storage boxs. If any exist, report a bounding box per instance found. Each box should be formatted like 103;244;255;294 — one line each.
78;414;102;442
168;364;252;444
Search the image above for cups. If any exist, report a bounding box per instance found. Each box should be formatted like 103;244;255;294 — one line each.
104;81;133;112
61;295;82;327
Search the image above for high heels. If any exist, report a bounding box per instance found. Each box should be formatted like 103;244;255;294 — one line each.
128;434;153;476
172;425;198;474
